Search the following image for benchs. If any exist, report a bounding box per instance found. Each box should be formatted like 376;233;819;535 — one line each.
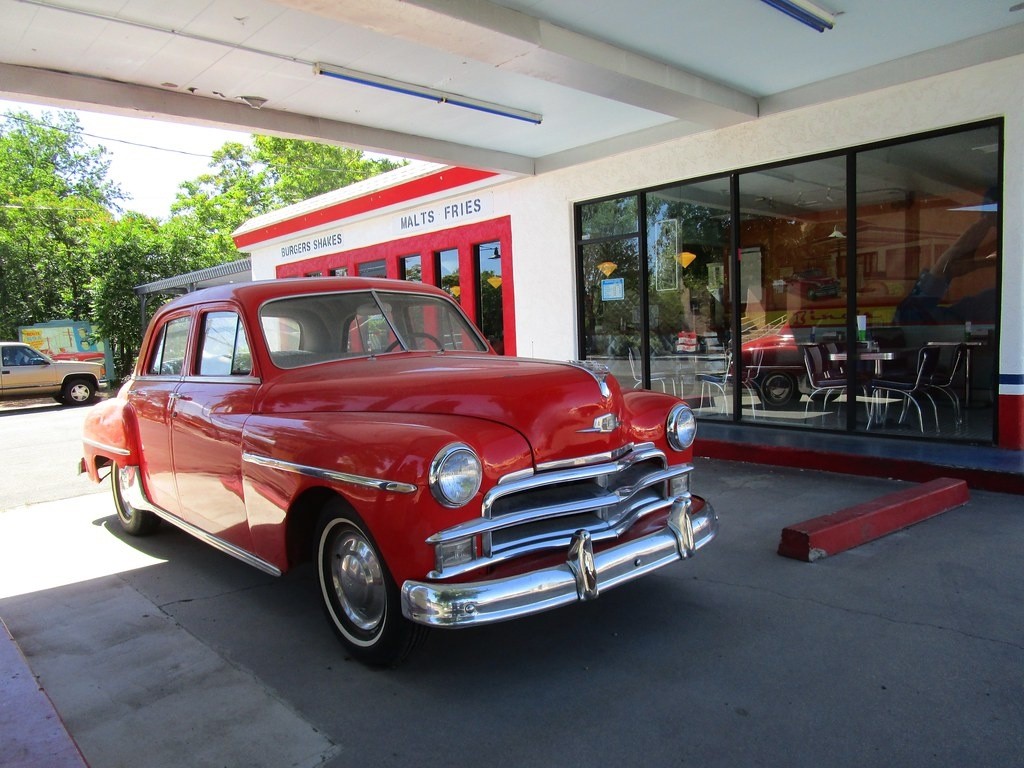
238;348;373;370
155;353;240;376
864;327;917;382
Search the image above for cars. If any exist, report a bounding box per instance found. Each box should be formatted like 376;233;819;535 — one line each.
0;341;108;408
77;275;720;668
722;297;958;412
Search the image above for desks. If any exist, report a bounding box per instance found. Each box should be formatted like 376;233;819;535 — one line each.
828;345;918;431
925;340;992;412
651;354;713;400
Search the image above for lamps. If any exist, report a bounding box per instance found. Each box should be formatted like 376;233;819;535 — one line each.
673;252;696;269
450;286;460;297
597;261;618;279
827;230;847;280
312;62;543;126
760;0;837;33
486;275;502;289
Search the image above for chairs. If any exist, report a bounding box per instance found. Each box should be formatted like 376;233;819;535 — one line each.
627;341;968;435
